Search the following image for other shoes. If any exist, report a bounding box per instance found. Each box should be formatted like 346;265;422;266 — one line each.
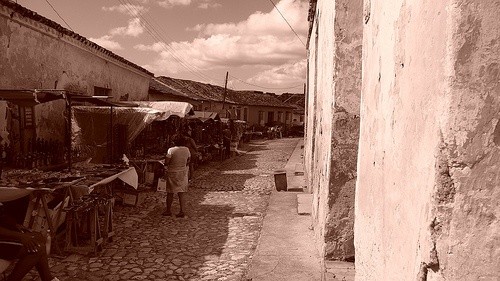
176;213;184;217
51;277;60;281
163;212;171;216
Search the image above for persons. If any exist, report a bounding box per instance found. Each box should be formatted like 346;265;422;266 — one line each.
227;119;240;156
162;135;190;217
223;125;231;160
0;226;59;281
292;119;300;137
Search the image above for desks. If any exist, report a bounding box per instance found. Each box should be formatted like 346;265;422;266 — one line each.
0;158;165;258
